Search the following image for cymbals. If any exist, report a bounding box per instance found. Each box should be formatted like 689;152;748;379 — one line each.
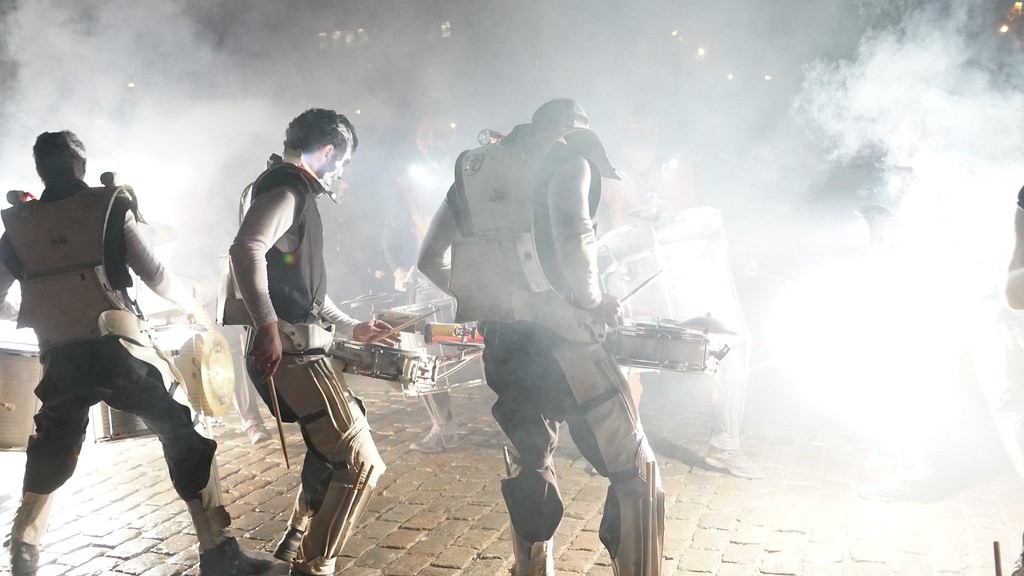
200;330;235;418
338;288;390;308
145;307;185;317
670;318;735;333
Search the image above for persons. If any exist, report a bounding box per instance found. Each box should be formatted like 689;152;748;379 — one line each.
0;129;281;575
416;98;668;576
374;155;464;450
1005;185;1023;309
216;107;405;576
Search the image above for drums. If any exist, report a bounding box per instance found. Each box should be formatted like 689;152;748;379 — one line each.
0;341;45;451
372;298;488;393
603;322;730;376
88;324;212;445
334;338;438;391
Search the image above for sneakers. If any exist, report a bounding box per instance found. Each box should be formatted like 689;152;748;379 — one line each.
3;531;41;576
248;423;273;445
273;525;304;563
199;538;274;576
409;431;459;454
